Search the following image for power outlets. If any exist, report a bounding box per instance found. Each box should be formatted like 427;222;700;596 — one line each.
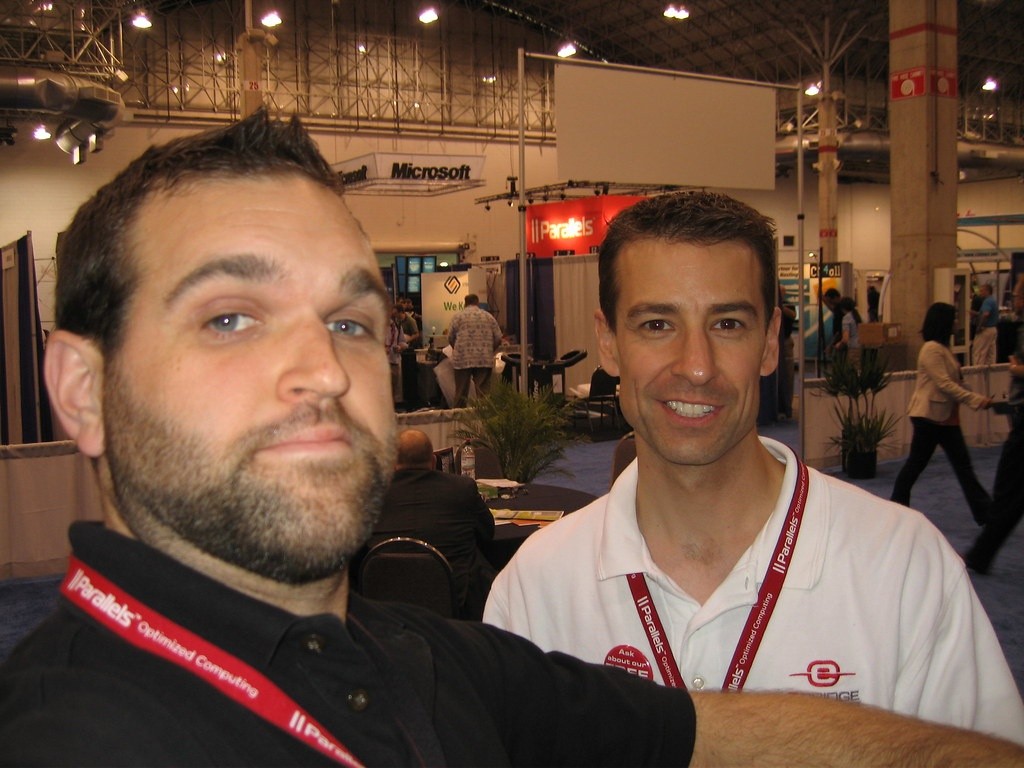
477;264;502;275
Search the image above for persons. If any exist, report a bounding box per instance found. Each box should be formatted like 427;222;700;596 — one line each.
0;109;1024;768
482;191;1024;747
366;275;1024;620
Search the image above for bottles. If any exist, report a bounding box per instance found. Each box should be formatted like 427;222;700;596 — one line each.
460;438;475;480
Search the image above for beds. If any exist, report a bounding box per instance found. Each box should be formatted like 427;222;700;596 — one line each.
433;447;455;474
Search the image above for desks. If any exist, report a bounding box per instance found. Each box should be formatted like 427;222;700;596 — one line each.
477;482;601;571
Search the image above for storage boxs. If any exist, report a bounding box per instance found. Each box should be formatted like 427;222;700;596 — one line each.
413;348;442;364
427;335;450;349
856;322;911;372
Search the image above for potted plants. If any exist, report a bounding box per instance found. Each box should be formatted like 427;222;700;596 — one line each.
807;341;910;479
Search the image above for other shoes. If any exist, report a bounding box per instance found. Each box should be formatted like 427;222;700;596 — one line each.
956;551;986;574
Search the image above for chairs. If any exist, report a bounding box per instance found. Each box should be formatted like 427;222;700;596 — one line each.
455;437;503;480
569;364;621;442
357;535;455;620
